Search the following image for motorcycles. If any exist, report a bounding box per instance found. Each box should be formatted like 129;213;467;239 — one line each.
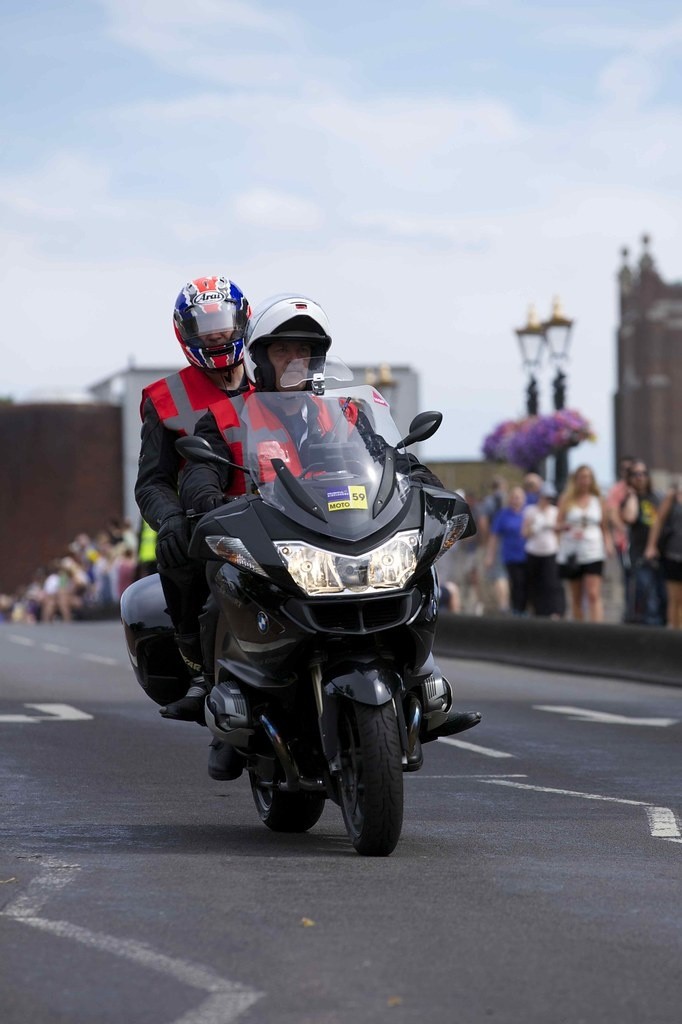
117;352;477;859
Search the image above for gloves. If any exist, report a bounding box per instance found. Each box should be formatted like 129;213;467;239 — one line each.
154;509;198;572
180;468;227;514
406;465;445;491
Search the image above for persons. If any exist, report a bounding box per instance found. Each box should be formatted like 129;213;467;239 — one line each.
134;275;253;728
435;455;682;629
178;294;481;781
0;516;158;626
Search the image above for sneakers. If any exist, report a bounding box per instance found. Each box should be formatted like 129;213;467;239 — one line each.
158;682;211;721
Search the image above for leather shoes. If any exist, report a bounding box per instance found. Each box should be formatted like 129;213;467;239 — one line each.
402;739;424;772
208;739;244;781
418;706;482;744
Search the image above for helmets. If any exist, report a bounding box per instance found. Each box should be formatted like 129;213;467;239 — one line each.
244;294;333;394
172;273;254;372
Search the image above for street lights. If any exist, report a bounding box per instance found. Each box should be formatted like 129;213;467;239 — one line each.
516;294;575;502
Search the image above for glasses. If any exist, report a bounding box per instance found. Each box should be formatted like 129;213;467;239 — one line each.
630;471;648;477
